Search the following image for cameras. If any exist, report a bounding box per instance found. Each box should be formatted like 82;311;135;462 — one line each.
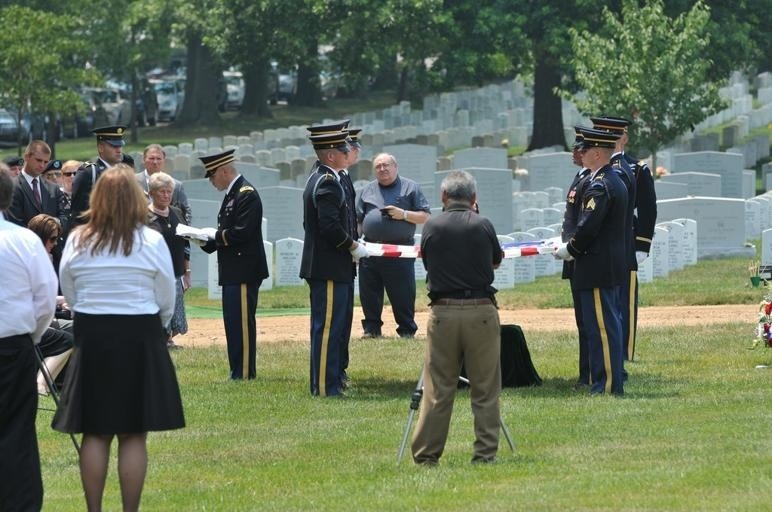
380;209;389;216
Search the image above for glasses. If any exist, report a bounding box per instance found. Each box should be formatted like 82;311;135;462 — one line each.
49;236;59;243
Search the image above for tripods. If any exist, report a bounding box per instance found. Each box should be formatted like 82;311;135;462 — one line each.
33;336;81;456
396;364;517;460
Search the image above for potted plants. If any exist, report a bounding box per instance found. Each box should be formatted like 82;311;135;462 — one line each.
748;258;761;289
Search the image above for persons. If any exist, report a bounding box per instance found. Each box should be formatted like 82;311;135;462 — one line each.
355;153;432;340
409;169;504;468
553;116;658;396
190;148;270;381
300;117;368;399
1;167;58;511
0;124;191;396
51;164;186;511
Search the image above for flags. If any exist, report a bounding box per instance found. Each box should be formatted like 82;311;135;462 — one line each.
360;237;557;259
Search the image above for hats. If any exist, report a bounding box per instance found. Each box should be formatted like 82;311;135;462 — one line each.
307;119;362;152
199;149;235;178
570;117;631;149
2;126;126;176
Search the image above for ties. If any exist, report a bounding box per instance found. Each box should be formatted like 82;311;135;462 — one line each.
31;179;42;208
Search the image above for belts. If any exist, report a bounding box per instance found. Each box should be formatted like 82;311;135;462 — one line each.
433;298;492;306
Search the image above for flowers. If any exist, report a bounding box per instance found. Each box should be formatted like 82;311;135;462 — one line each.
749;298;772;350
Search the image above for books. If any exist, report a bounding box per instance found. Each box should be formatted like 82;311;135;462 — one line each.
174;222;215;242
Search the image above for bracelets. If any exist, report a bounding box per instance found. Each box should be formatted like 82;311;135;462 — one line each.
403;209;408;222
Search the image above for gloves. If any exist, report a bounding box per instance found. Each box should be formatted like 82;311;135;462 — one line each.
201;228;217;240
350;244;367;258
553;243;570;259
637;251;646;263
